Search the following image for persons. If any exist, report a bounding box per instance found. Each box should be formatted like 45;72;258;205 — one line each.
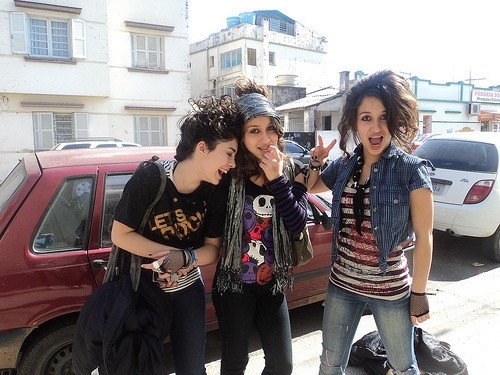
110;94;245;375
212;76;308;375
305;69;436;375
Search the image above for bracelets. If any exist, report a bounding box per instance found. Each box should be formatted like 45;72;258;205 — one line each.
309;161;321;171
182;246;196;268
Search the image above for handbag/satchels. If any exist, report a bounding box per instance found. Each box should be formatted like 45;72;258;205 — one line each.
288;154;314;269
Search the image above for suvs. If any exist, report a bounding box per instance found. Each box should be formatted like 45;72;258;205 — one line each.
49;137;144;203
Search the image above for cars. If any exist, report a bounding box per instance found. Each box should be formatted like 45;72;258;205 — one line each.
284;140;313;178
35;232;54;249
408;131;500;263
0;146;415;375
